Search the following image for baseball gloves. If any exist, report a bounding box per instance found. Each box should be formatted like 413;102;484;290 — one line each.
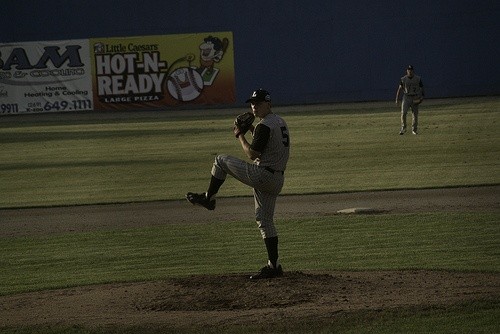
413;96;423;105
233;112;255;137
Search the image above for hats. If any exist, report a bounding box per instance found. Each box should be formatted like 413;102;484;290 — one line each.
406;64;413;70
245;90;271;104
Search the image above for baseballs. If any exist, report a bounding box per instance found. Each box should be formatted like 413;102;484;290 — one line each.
167;68;204;102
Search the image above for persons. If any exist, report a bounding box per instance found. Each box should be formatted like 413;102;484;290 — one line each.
396;64;425;135
184;89;290;280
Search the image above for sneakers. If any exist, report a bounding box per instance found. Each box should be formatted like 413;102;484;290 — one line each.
249;264;283;279
399;128;406;135
185;192;216;210
411;129;418;135
